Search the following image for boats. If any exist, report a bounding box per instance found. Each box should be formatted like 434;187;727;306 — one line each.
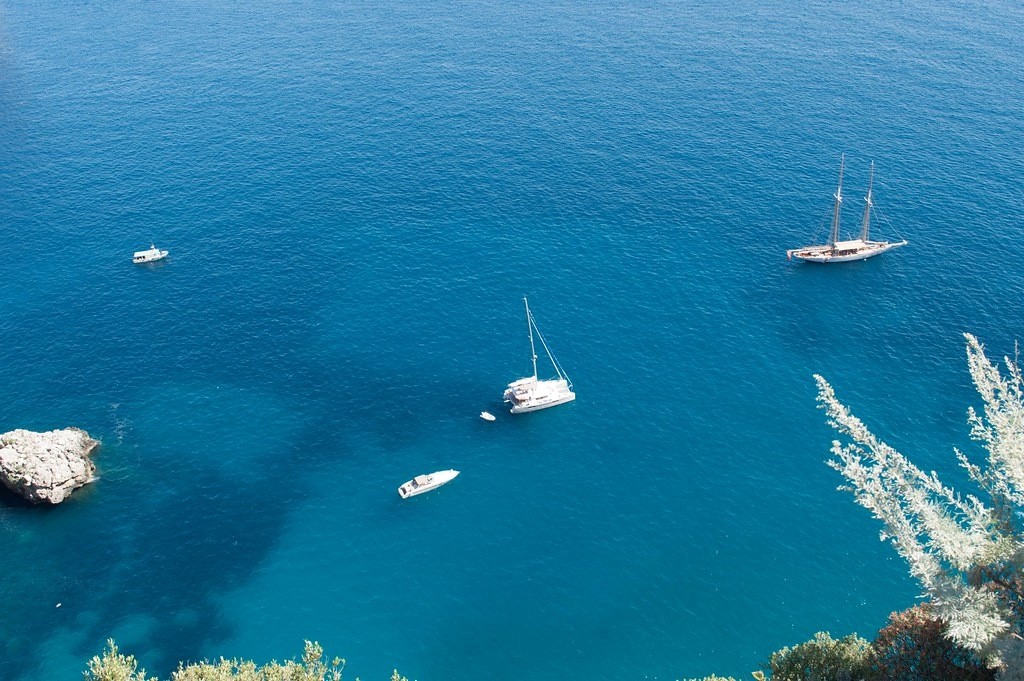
133;245;168;263
480;412;496;421
398;469;459;499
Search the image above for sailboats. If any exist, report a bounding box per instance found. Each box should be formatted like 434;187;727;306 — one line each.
504;298;575;414
786;154;908;262
